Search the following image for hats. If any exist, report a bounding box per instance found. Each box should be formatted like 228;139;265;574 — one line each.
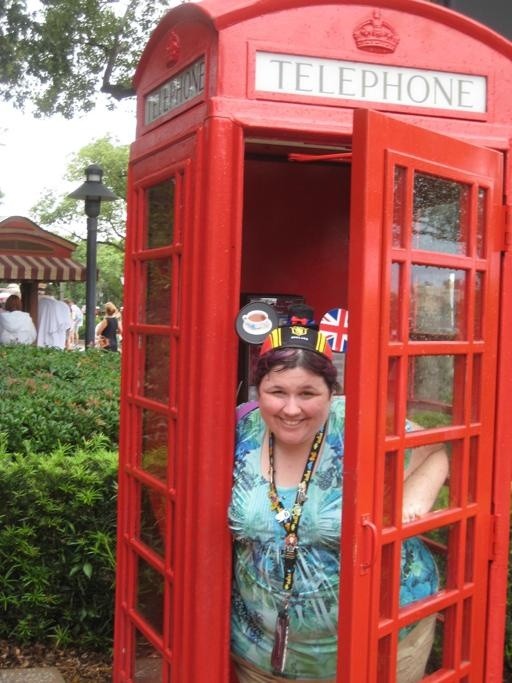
258;326;333;362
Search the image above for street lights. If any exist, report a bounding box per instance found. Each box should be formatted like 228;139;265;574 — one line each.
65;163;119;350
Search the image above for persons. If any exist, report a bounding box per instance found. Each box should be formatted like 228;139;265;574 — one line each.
1;284;122;352
224;348;450;683
